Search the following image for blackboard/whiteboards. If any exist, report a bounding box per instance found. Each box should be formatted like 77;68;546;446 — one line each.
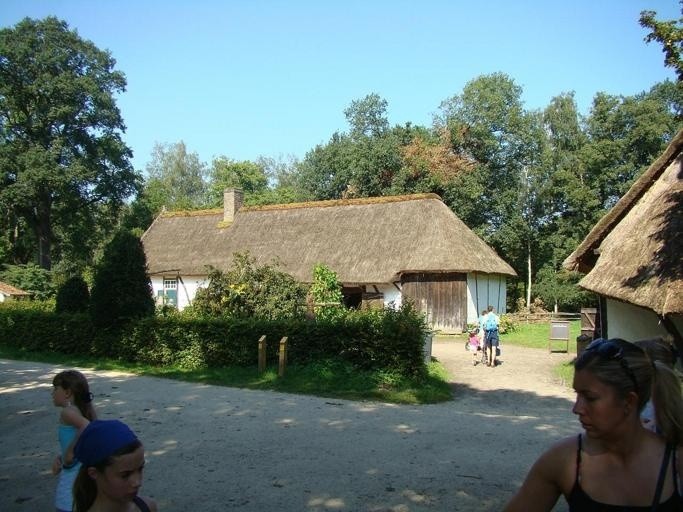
550;321;570;340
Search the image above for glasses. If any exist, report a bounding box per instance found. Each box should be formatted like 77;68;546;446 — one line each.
583;337;639;391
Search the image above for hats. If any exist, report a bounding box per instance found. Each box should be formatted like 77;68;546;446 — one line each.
74;420;135;467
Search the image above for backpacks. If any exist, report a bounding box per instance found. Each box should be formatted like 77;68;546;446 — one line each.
484;313;498;339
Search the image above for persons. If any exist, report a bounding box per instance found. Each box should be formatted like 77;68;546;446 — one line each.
73;419;157;511
635;340;678;437
504;338;682;512
478;311;488;364
469;332;480;366
50;370;97;512
482;306;499;367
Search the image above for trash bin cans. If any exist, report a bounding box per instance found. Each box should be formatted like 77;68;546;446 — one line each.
422;336;432;363
